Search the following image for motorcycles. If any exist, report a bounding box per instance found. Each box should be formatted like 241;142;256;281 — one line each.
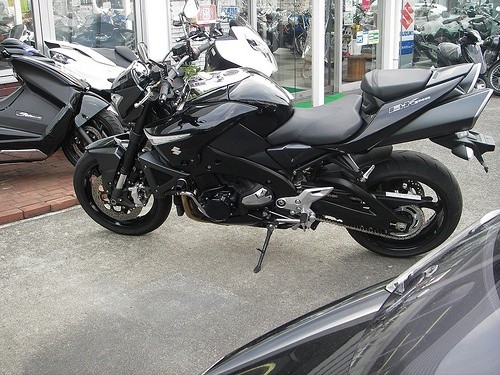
72;1;497;273
413;7;500;94
66;17;137;50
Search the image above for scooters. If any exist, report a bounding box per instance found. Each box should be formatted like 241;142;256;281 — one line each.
0;16;142;170
